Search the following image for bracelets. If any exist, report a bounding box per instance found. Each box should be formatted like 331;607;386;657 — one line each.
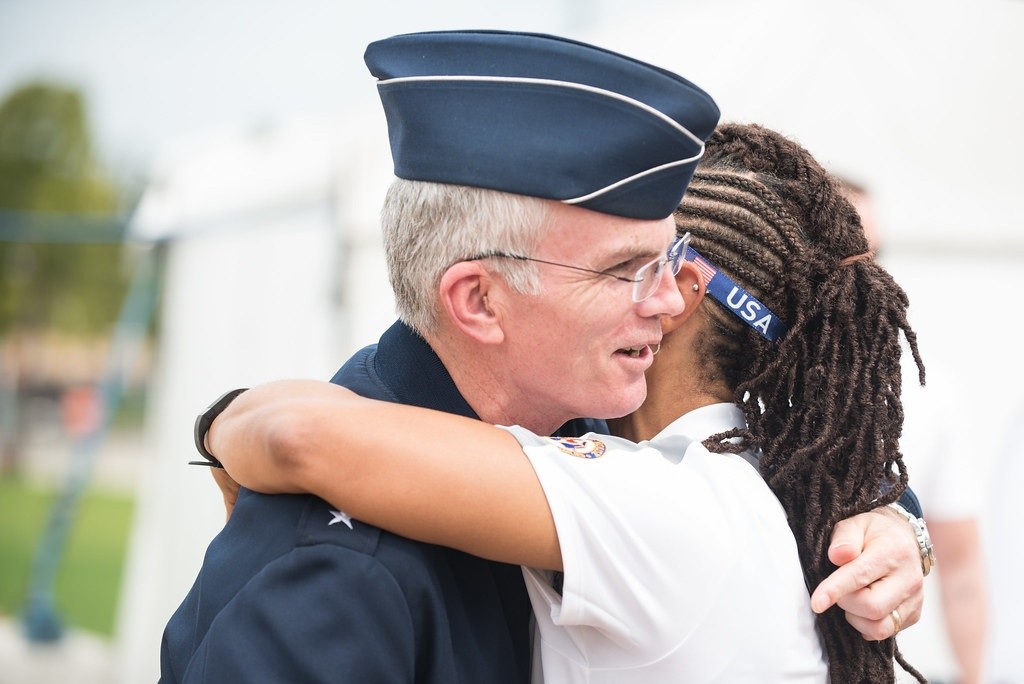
188;388;251;469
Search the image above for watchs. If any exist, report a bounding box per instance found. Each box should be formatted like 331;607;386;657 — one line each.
887;501;936;576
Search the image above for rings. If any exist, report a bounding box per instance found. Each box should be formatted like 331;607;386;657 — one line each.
890;609;902;636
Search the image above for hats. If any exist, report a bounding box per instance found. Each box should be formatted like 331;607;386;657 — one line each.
362;28;720;220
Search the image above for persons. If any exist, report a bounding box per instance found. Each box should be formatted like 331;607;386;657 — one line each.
162;29;927;684
829;175;994;684
191;117;926;684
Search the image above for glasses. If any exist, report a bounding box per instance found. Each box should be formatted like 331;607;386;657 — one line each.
445;231;695;303
